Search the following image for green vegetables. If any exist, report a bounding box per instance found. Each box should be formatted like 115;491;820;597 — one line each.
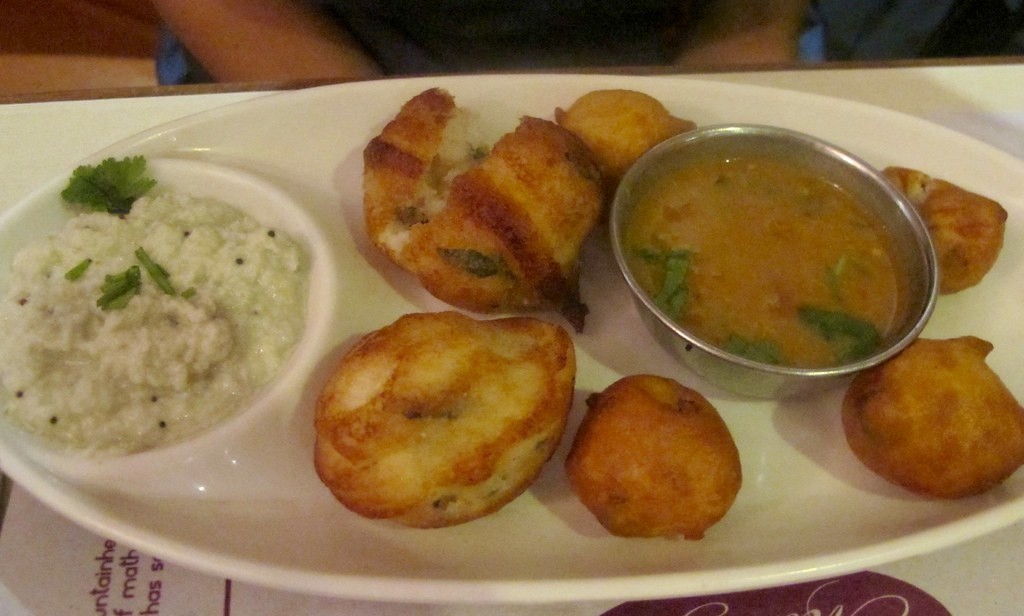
640;243;883;369
61;154;198;312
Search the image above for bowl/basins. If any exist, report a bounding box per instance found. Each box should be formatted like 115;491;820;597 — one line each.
612;124;940;399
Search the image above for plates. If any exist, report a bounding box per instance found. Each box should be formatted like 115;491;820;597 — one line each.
2;71;1024;604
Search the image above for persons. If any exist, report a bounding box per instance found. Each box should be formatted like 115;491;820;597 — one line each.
152;0;823;83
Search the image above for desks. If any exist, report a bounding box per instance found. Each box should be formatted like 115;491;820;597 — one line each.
0;55;1024;615
